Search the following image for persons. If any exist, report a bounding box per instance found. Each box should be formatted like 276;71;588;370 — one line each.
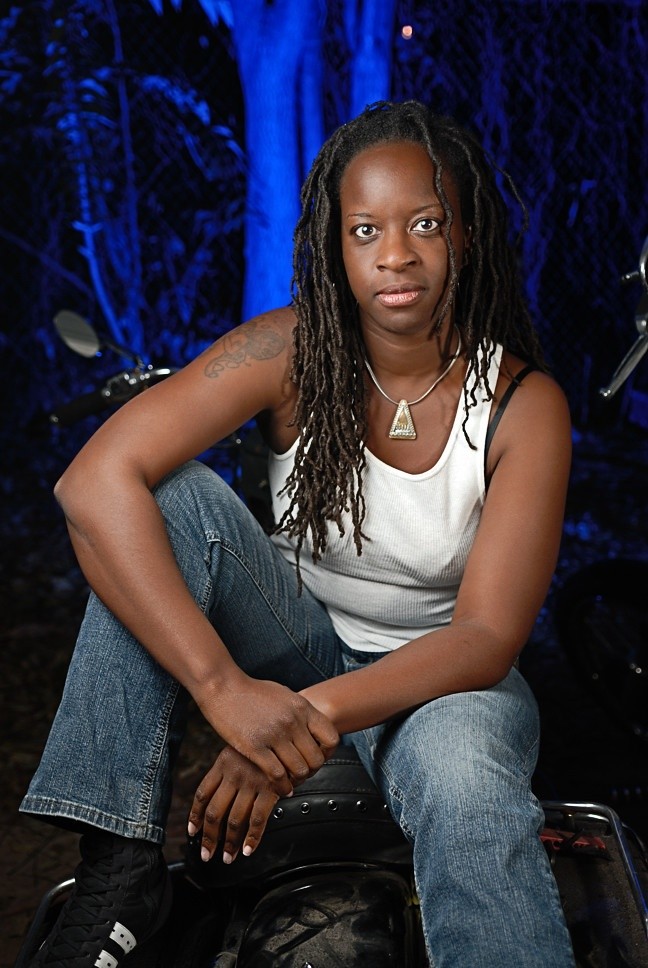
9;97;579;968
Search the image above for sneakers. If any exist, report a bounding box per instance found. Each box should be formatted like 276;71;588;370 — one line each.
29;832;172;968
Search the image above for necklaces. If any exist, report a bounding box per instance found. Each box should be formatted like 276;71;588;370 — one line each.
363;325;462;440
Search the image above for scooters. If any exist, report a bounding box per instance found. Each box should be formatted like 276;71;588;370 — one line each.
19;310;647;967
